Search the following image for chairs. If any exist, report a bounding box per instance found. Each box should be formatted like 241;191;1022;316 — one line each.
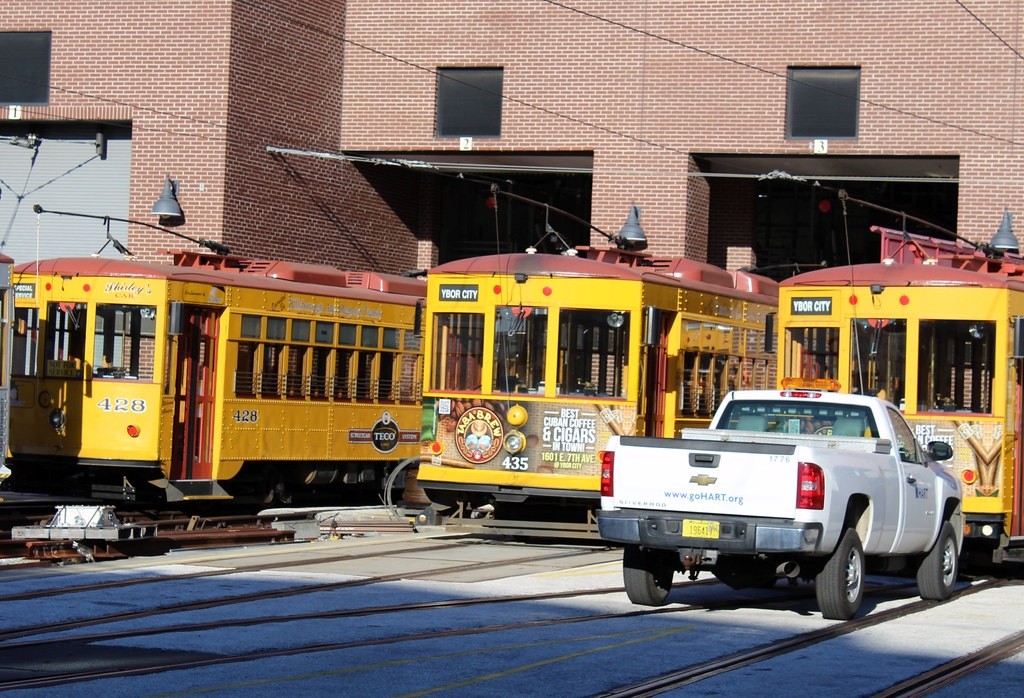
737;415;768;432
832;417;865;437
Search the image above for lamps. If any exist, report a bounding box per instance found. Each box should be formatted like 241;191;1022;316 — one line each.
990;209;1019;252
618;204;647;244
151;175;182;219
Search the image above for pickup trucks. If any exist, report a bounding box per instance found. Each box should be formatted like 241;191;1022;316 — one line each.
595;377;967;620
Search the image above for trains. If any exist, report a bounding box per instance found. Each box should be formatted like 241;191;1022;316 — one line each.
415;243;781;526
777;225;1024;576
7;247;432;514
